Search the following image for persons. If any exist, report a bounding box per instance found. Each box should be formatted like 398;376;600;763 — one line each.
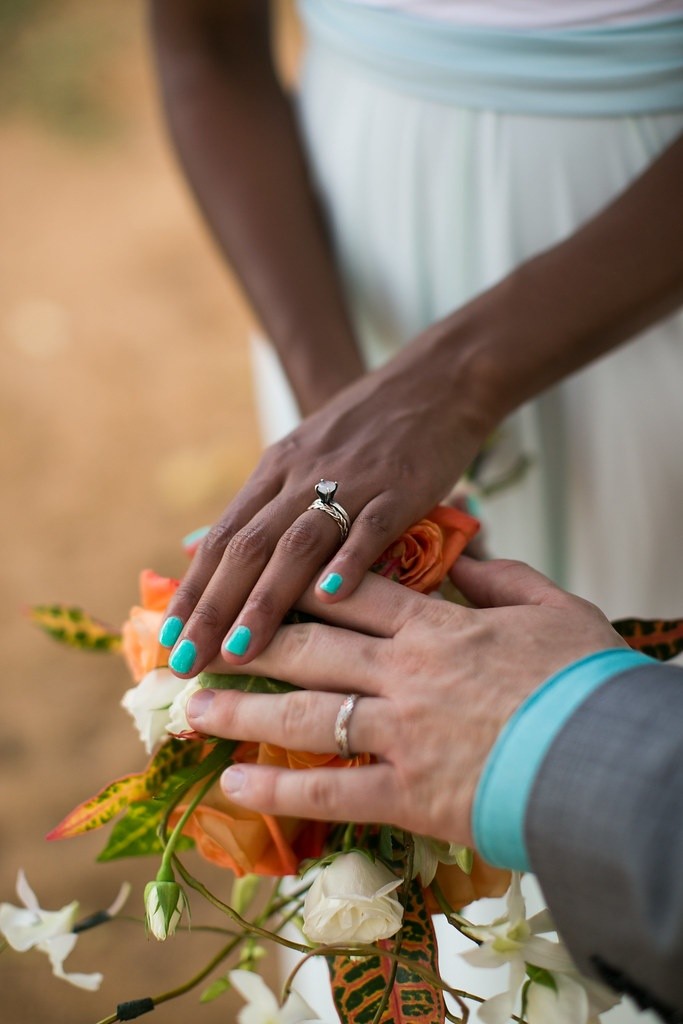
184;550;683;1023
156;2;683;682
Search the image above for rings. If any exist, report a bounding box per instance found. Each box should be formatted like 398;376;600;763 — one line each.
308;478;351;542
333;692;362;760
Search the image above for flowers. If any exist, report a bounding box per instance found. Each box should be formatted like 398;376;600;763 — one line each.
0;504;683;1024
